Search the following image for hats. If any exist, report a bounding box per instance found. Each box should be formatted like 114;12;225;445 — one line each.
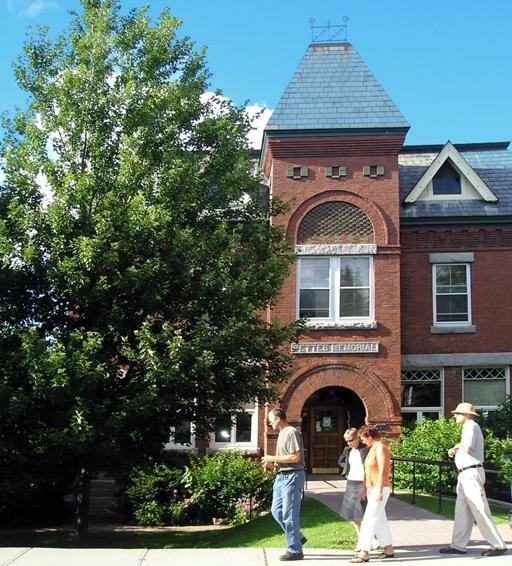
451;403;480;417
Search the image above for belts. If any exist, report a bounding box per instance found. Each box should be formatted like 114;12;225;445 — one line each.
458;465;483;472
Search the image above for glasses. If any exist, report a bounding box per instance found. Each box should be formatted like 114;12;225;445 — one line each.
345;439;354;443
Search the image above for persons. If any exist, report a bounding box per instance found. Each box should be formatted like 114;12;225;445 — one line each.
439;402;508;556
348;424;395;562
260;407;308;562
338;427;380;553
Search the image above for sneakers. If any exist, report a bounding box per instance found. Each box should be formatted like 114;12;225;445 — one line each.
279;551;303;560
439;546;466;554
301;536;307;544
482;548;507;556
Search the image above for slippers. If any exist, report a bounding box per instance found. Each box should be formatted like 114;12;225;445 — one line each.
379;553;394;558
350;557;369;563
